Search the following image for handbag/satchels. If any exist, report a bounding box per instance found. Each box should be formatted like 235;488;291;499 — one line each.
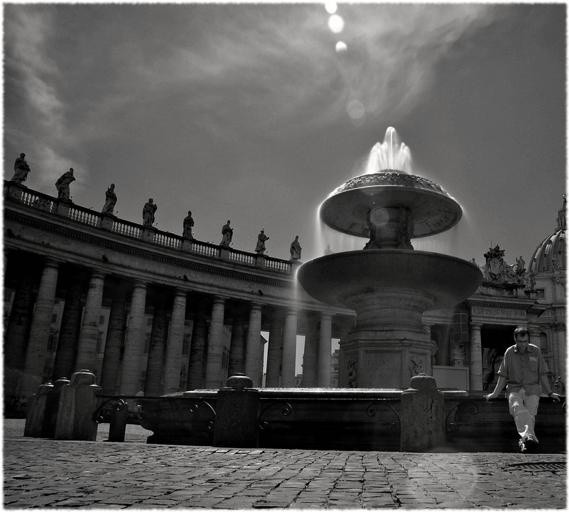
548;392;554;397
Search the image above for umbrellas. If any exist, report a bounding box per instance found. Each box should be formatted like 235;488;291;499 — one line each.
518;438;538;453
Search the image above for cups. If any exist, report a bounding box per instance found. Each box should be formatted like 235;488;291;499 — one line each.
517;340;528;343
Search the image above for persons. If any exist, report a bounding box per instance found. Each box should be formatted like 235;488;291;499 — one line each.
7;152;32;185
254;227;271;255
102;182;119;218
482;326;562;455
218;219;234;248
182;210;195;239
143;196;157;228
514;254;525;273
290;234;303;263
53;166;77;201
